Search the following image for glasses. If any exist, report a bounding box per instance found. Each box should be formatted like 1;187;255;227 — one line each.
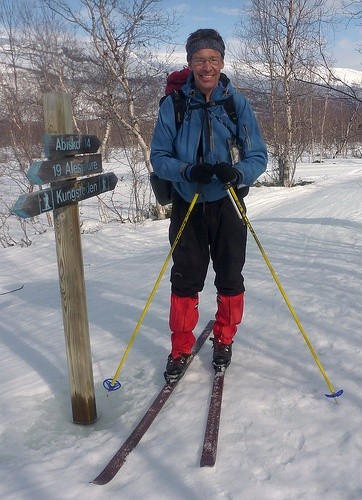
191;58;223;65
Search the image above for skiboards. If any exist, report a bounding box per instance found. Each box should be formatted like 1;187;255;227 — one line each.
88;314;226;487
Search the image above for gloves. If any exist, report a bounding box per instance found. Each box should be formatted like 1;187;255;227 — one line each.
190;162;213;183
213;161;236;183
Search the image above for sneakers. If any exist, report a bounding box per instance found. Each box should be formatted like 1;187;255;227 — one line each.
209;337;234;371
166;349;192;378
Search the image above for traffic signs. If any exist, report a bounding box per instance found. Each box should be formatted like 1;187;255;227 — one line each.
11;172;119;220
27;156;104;184
45;132;101;156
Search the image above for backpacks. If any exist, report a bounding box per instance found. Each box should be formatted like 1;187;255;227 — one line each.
149;66;245;206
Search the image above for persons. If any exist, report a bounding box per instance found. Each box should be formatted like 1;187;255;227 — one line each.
150;27;268;381
